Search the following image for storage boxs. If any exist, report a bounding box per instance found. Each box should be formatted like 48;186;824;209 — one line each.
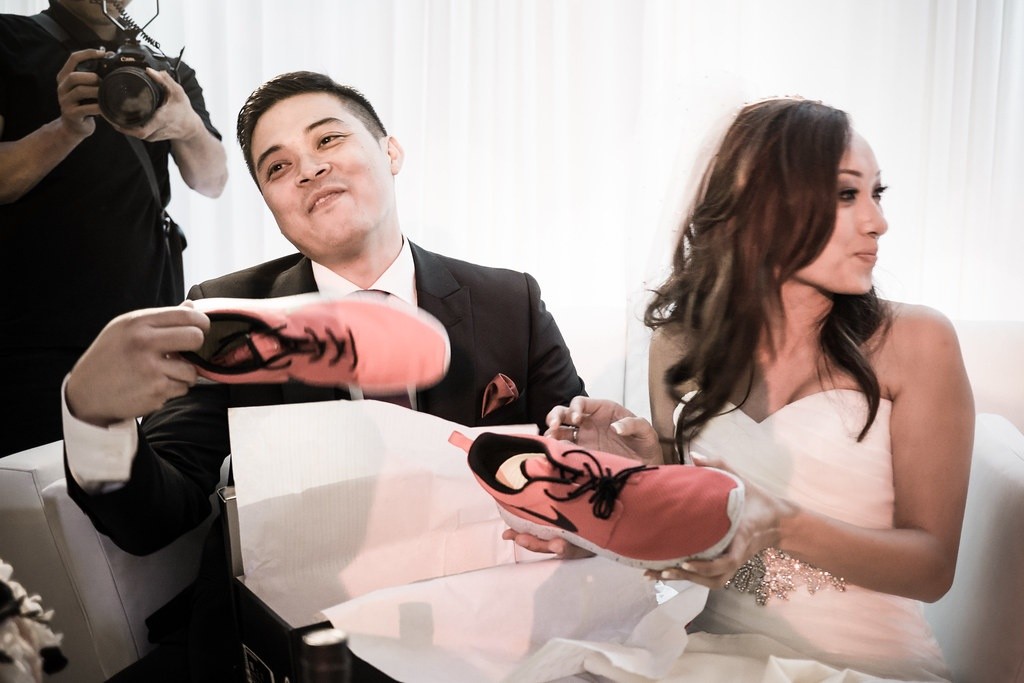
232;574;395;683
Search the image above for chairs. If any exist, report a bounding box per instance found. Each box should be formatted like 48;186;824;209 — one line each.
624;269;1024;682
0;440;231;683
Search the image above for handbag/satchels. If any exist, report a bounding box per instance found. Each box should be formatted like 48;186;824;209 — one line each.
155;210;188;305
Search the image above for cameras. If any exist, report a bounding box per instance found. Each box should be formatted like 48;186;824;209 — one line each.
76;41;182;128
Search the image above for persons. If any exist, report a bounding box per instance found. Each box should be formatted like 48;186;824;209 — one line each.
543;95;977;683
61;69;596;683
0;0;229;456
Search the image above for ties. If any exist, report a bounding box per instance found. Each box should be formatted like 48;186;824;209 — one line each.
360;387;412;410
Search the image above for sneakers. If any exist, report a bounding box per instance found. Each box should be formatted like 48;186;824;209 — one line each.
448;429;745;570
166;293;451;394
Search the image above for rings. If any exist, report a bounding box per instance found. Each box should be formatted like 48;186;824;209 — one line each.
572;425;579;439
